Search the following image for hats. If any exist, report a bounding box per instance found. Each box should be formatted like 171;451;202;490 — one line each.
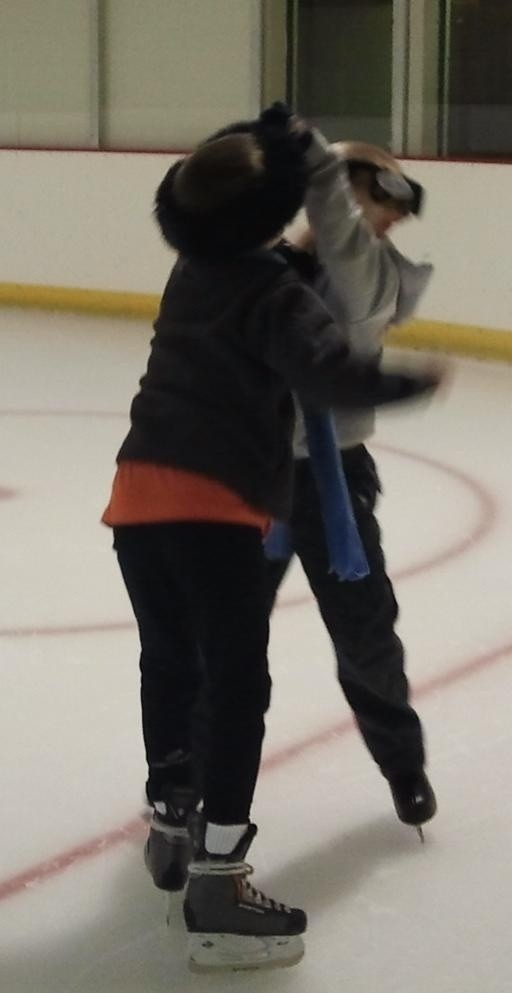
331;140;421;217
156;115;306;261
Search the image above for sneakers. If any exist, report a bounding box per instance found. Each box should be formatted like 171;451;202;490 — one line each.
143;784;200;889
390;772;435;824
183;813;306;935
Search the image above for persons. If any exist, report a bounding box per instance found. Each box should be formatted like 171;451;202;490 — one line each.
188;101;436;854
103;123;450;937
